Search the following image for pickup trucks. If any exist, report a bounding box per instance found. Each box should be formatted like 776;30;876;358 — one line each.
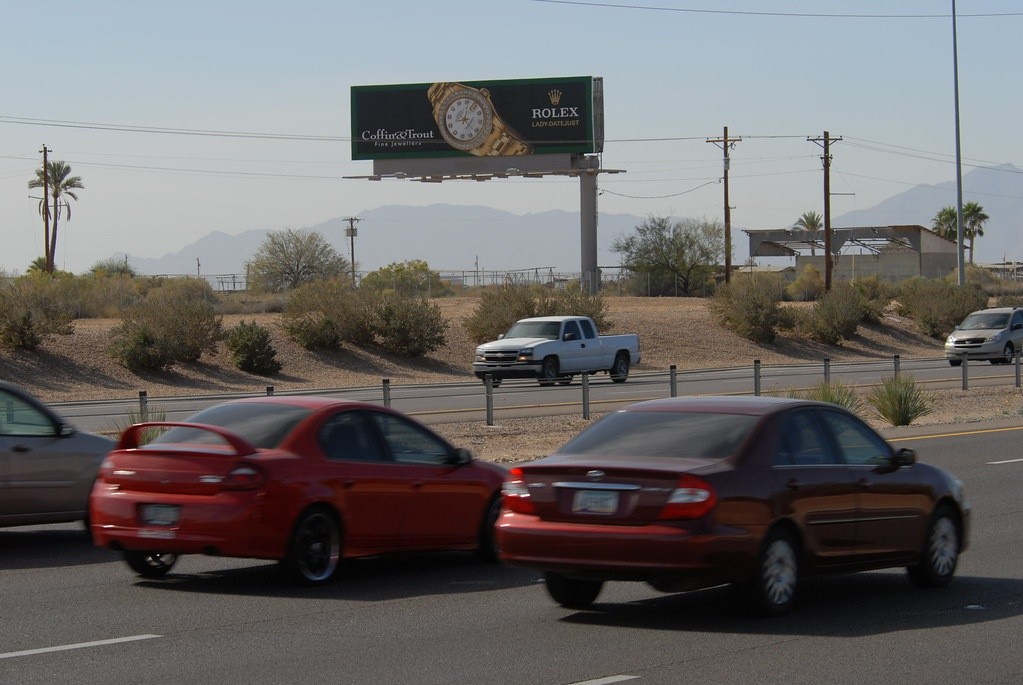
473;316;643;387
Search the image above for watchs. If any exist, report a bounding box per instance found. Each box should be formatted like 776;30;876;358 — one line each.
427;82;534;157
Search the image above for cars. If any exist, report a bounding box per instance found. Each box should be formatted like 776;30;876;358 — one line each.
93;395;518;587
0;377;126;546
944;307;1023;366
495;395;977;618
